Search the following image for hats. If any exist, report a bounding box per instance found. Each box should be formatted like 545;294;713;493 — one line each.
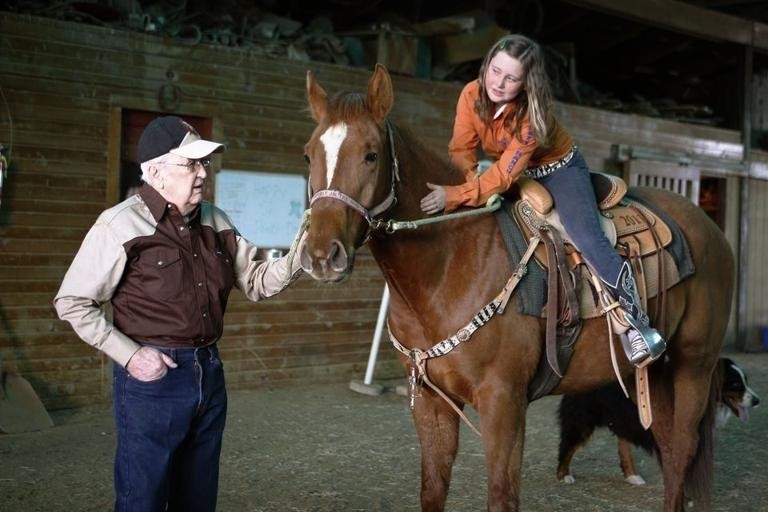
137;115;227;164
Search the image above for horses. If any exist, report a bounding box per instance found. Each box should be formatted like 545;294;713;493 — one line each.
297;62;735;512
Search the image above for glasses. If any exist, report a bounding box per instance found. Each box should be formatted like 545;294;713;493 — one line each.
159;158;211;169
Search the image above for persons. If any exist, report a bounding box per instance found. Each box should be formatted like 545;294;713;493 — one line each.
50;116;308;512
418;33;665;369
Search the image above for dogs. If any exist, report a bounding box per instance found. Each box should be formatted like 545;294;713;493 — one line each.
554;357;761;486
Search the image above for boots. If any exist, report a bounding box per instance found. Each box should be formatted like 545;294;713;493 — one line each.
598;261;659;365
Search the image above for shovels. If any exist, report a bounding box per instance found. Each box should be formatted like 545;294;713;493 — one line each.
0;365;56;435
